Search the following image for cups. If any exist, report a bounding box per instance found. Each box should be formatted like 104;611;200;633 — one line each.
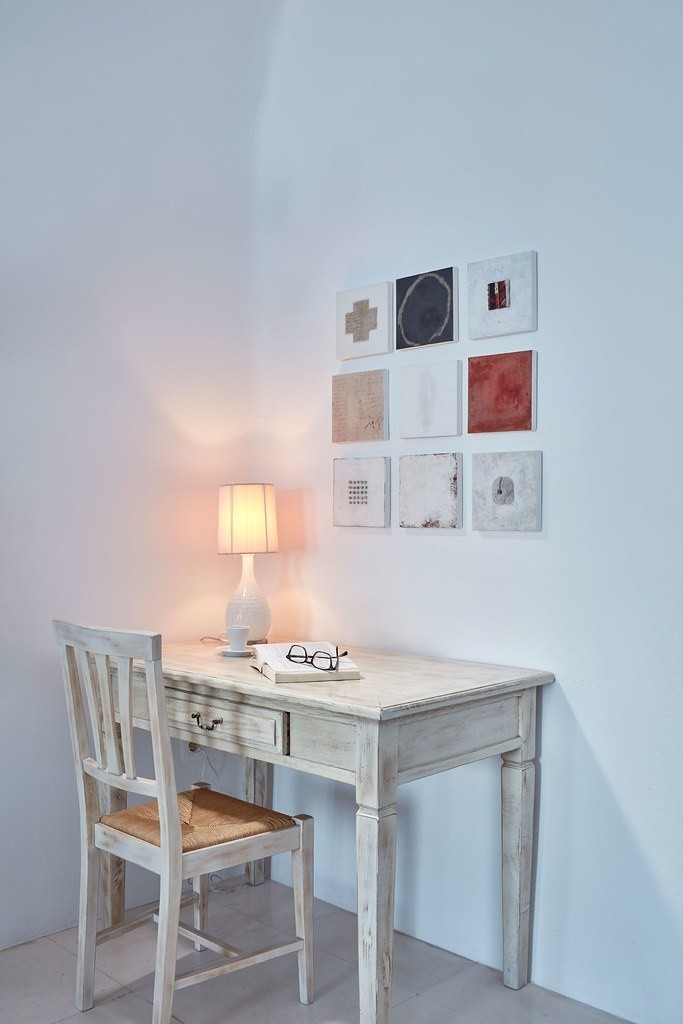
219;625;250;651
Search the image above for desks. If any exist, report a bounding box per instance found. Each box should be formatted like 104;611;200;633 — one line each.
96;635;556;1024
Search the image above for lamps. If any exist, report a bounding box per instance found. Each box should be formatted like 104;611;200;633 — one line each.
216;483;281;643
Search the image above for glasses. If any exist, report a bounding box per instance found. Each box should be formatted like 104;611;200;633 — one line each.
285;645;349;672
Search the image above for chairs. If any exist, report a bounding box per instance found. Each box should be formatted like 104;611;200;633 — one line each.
49;618;316;1024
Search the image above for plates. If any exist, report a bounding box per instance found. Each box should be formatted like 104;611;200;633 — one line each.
215;646;255;657
